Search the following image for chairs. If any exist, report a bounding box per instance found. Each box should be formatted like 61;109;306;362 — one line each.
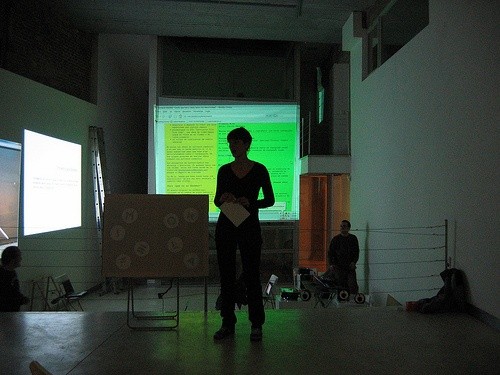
261;273;280;309
53;273;86;312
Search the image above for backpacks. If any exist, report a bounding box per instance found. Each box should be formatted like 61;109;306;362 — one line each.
437;268;469;305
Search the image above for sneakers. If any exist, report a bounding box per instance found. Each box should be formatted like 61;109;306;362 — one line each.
250;325;263;341
213;324;236;339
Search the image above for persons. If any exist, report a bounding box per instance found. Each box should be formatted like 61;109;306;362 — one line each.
324;219;362;304
0;245;31;314
212;126;275;340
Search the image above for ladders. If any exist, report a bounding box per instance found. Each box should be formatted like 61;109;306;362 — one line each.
87;125;122;297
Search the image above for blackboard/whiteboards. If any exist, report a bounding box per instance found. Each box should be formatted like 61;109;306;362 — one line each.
102;193;209;278
